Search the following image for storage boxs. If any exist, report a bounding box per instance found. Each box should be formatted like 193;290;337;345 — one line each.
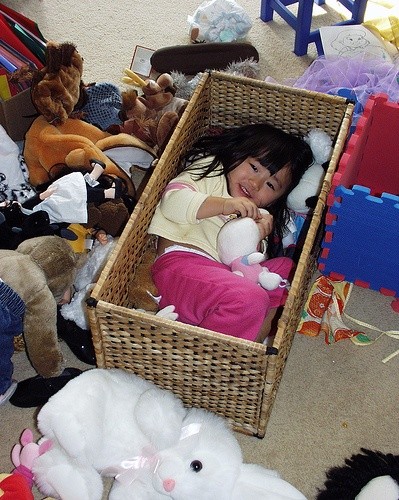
85;67;356;440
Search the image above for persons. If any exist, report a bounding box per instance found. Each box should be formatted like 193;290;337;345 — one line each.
148;122;296;346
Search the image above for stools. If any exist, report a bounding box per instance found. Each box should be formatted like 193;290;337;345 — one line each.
260;0;368;57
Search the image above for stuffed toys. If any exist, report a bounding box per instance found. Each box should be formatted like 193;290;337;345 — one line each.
129;128;334;321
0;43;188;404
0;367;399;500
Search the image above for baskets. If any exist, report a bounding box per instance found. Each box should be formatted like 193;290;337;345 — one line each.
85;69;356;440
